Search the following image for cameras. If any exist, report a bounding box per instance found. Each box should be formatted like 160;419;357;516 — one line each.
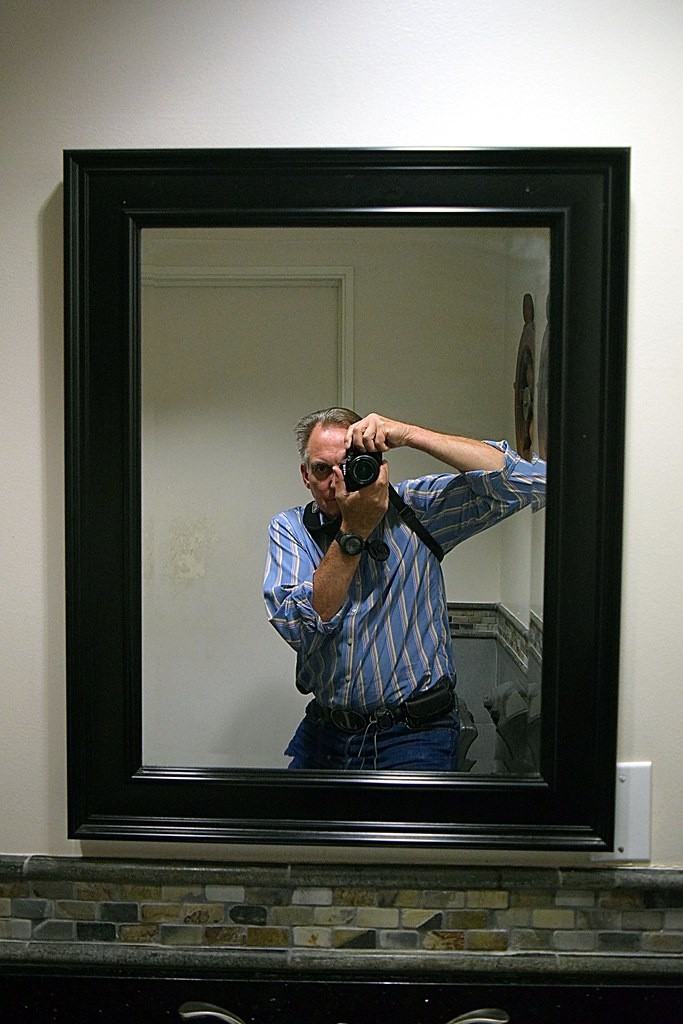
341;442;383;492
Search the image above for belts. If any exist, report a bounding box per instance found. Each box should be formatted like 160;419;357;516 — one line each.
315;701;404;734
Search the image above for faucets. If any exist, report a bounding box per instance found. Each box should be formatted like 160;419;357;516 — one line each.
178;1001;244;1024
447;1007;510;1024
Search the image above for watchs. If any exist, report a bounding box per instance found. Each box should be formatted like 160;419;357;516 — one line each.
335;530;364;556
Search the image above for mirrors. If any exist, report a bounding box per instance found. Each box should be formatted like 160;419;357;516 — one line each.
63;147;631;853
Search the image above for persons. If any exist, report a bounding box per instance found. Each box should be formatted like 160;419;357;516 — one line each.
261;407;532;771
